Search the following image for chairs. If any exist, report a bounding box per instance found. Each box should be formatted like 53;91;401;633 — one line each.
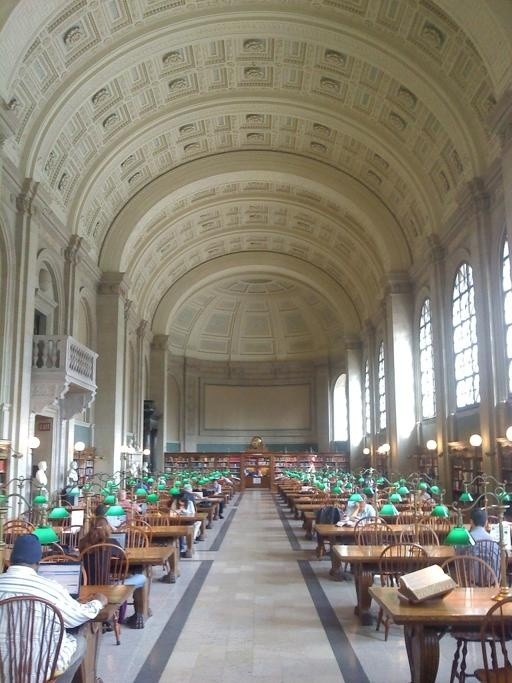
438;556;508;683
1;463;240;629
273;465;512;626
376;543;430;642
0;596;64;683
78;542;129;645
474;595;511;682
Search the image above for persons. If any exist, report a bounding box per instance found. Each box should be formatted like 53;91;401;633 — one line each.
0;534;108;683
80;518;146;633
170;479;224;552
69;461;78;481
456;508;502;587
36;461;47;485
344;500;379;521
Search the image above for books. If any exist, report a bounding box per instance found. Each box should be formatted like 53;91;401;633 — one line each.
165;456;347;473
490;524;511;548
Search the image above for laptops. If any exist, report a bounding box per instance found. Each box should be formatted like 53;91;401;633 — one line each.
109;532;127;557
106;515;128;528
36;562;82;602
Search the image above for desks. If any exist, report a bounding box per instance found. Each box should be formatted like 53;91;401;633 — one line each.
366;586;512;683
75;584;136;683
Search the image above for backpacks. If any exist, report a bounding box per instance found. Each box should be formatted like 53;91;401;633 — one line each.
317;507;343;523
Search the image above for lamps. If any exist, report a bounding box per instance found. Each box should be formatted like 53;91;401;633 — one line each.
75;440;85;459
426;439;437;465
469;433;482;466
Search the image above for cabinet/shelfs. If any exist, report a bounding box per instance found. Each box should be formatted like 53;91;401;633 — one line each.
164;451;347;488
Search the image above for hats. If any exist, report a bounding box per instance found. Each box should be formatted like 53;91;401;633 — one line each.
10;534;41;563
